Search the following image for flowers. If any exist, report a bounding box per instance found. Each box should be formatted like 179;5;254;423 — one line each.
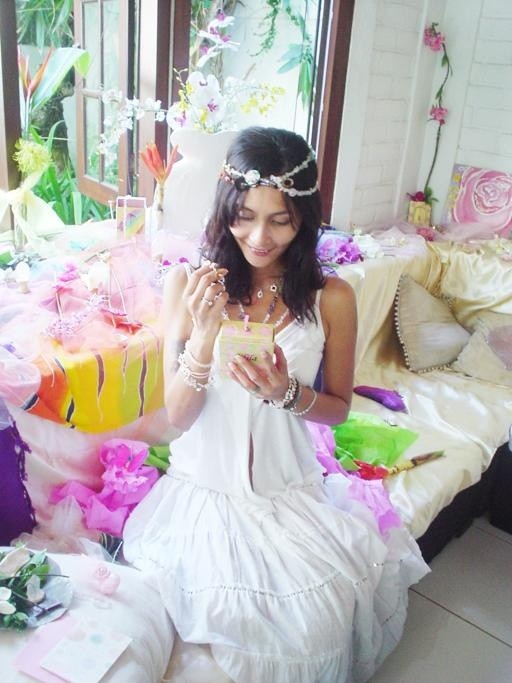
404;21;456;246
11;0;284;187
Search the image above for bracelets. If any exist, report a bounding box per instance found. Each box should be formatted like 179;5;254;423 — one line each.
178;341;216;391
269;375;317;416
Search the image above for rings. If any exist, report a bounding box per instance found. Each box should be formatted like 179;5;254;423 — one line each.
209;263;226;295
202;297;213;306
250;388;259;395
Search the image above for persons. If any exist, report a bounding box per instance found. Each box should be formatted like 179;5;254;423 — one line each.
121;125;433;683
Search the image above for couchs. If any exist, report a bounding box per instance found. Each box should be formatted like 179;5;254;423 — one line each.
2;248;512;683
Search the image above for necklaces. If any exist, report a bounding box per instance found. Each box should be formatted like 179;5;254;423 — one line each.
222;269;293;329
